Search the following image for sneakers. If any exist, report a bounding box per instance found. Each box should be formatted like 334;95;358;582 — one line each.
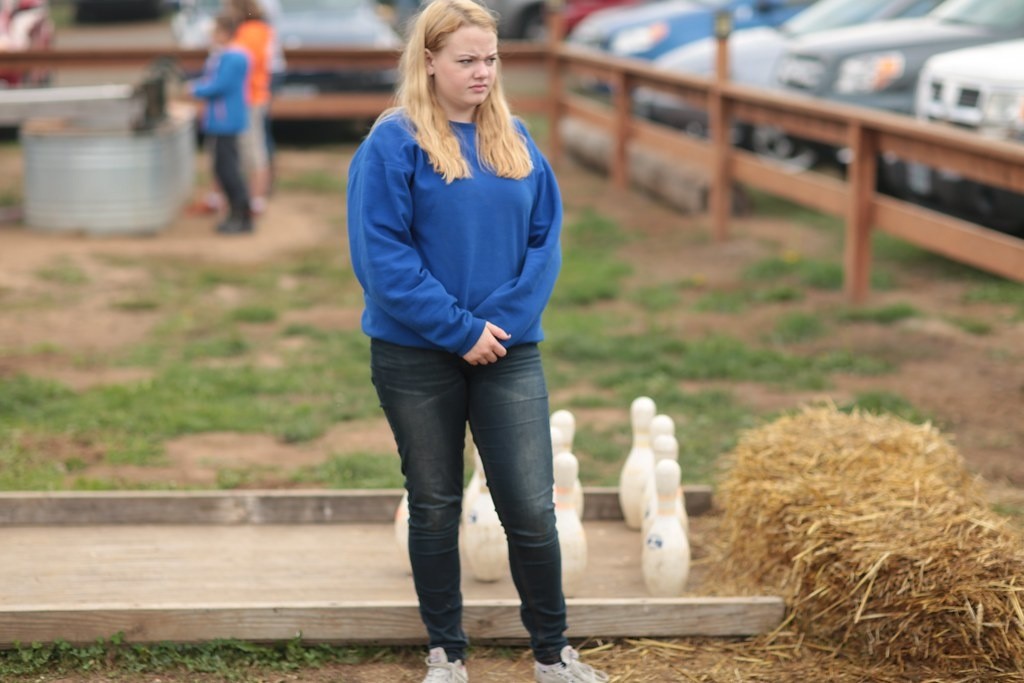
533;645;609;683
421;646;469;683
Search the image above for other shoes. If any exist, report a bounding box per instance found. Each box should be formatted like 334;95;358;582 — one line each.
219;216;252;232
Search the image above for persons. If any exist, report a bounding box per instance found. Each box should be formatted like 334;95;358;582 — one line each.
181;13;254;234
345;0;610;683
184;0;287;219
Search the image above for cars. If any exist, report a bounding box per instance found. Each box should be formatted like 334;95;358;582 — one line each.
171;1;404;130
483;3;1023;203
1;1;55;134
915;40;1023;232
